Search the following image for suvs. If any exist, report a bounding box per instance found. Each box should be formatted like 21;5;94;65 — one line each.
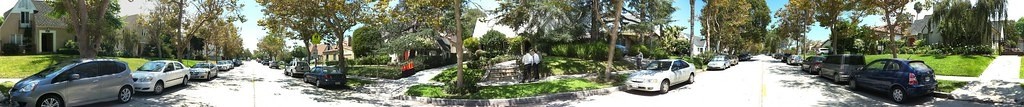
816;48;829;56
284;61;310;77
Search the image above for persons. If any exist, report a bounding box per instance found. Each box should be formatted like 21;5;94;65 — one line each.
518;49;541;83
637;49;645;70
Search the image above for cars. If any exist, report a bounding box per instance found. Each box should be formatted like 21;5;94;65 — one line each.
8;59;135;107
216;59;244;71
303;66;346;88
256;58;279;69
707;53;753;70
801;56;827;74
772;53;803;65
132;60;219;95
849;59;938;103
626;59;696;93
616;45;628;57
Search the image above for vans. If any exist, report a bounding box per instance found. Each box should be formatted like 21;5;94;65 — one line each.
819;54;866;83
